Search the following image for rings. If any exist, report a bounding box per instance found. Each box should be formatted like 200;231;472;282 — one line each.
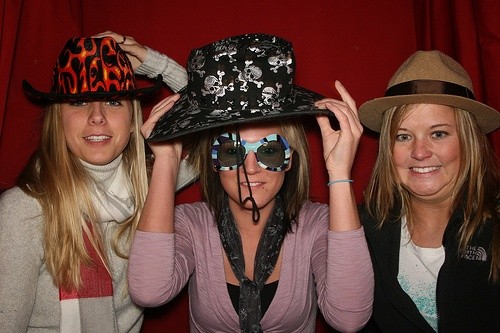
117;36;127;45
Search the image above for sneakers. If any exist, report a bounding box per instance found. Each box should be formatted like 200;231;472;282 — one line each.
358;50;500;134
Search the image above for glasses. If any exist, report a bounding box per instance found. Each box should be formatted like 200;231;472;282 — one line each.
211;132;291;172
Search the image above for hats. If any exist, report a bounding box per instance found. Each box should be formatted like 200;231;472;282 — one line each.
21;36;163;105
145;32;336;144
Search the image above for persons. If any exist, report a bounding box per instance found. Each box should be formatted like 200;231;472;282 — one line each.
0;30;189;333
126;33;375;333
356;49;497;333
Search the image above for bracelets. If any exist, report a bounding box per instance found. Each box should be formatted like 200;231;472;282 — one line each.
327;179;354;187
